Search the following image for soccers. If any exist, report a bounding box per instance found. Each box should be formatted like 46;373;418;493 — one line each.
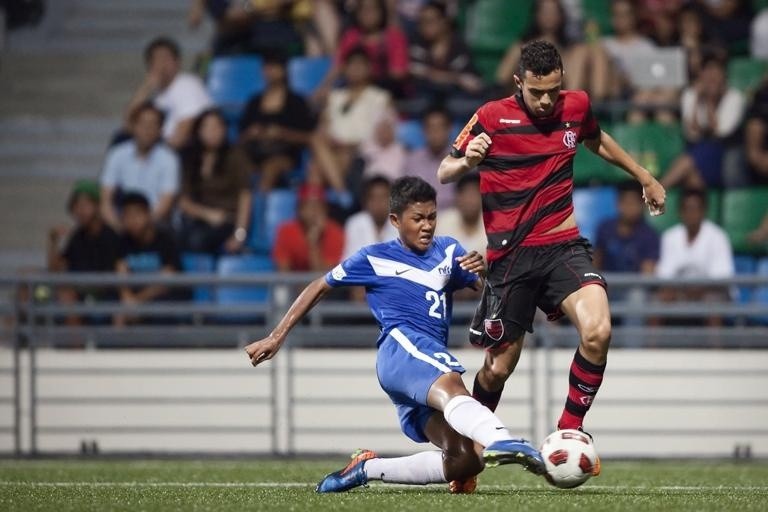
540;429;595;488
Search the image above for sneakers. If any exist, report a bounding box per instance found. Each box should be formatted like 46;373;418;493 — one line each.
448;474;479;494
316;449;378;492
557;423;601;475
481;437;546;475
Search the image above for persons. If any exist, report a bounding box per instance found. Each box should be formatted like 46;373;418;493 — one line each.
437;40;669;476
47;0;767;330
245;175;549;493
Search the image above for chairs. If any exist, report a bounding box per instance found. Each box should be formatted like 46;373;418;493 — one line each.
183;0;768;327
